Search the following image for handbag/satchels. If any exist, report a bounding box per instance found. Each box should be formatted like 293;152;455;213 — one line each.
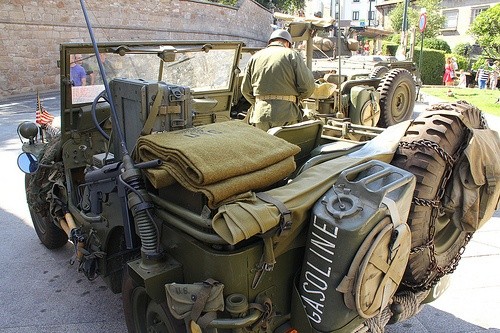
165;278;224;320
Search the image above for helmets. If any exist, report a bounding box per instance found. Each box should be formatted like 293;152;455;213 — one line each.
268;29;292;44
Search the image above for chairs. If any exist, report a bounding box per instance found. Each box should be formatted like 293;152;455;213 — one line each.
266;119;323;161
325;74;348;85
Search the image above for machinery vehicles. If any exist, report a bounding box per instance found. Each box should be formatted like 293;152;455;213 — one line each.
273;11;418;127
16;41;500;332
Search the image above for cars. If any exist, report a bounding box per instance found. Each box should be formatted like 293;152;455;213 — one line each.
470;56;500;88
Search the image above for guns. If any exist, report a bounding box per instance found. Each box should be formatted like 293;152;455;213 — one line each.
84;159;163;215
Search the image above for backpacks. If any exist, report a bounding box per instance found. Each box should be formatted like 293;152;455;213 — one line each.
421;111;500;231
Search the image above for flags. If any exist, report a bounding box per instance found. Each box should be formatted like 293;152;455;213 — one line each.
36;96;54;125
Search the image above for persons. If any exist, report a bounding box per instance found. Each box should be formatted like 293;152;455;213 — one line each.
69;52;119;85
475;58;499;89
241;28;316;131
363;39;370;55
443;57;466;88
